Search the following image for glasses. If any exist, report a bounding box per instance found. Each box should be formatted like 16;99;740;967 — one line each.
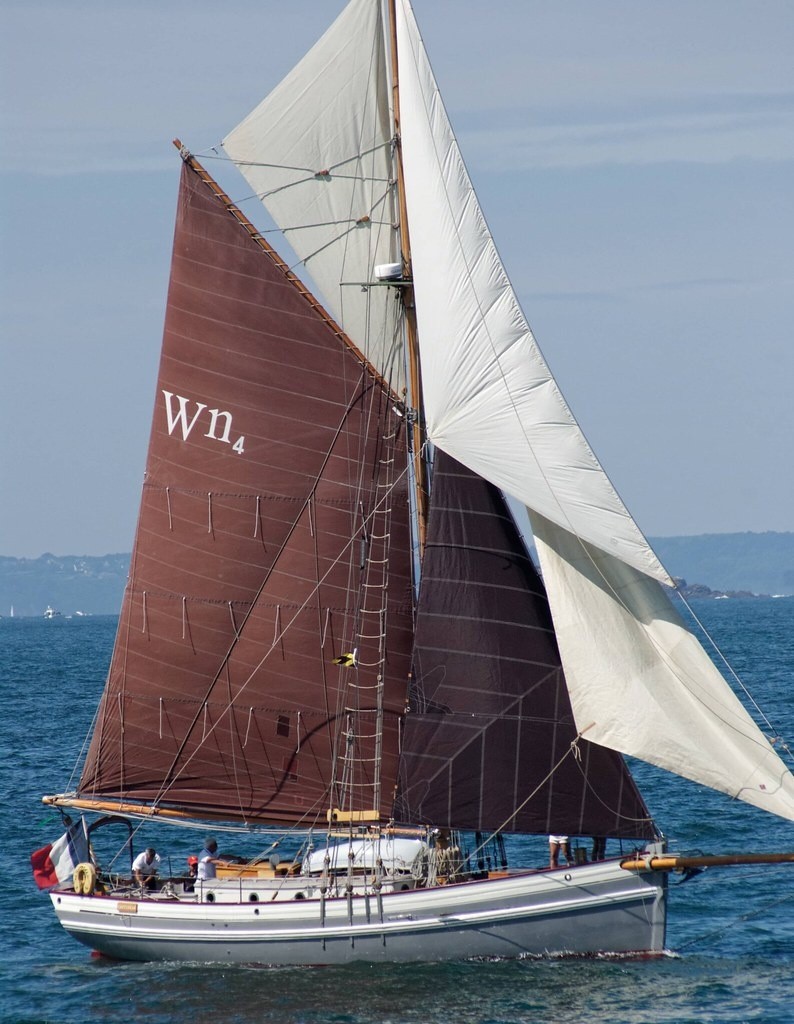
190;863;197;867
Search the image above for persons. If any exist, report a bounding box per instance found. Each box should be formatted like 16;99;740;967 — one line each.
548;835;574;869
132;847;161;894
181;838;224;893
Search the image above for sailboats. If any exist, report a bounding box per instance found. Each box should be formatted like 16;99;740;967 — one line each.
10;606;14;618
27;1;794;963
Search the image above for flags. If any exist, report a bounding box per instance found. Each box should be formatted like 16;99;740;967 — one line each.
29;820;90;890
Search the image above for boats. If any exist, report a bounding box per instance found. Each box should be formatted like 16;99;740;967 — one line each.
43;605;62;619
72;611;94;617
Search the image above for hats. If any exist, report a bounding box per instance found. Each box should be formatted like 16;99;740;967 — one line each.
188;856;198;866
